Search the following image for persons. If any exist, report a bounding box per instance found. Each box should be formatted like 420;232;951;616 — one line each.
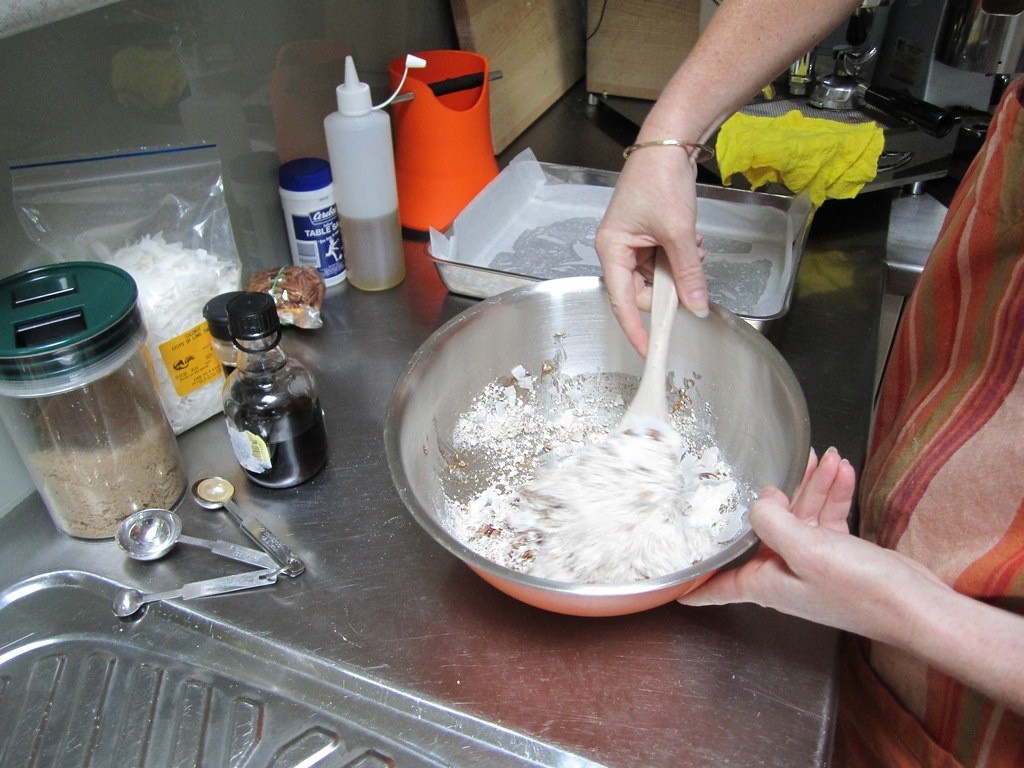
593;0;1024;768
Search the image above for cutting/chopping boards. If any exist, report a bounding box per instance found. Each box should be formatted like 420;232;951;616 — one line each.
447;0;705;156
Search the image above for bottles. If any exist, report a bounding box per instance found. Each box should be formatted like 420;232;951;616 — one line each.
279;158;347;290
221;295;329;489
0;258;190;543
323;55;406;291
202;291;243;378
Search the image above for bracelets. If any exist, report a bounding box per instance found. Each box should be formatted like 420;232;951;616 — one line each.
622;140;715;163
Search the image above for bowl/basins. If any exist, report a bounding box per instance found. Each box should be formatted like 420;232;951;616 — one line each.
381;275;812;618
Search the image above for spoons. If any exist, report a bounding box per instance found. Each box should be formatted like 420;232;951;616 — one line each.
191;477;306;574
114;508;306;578
112;568;278;618
